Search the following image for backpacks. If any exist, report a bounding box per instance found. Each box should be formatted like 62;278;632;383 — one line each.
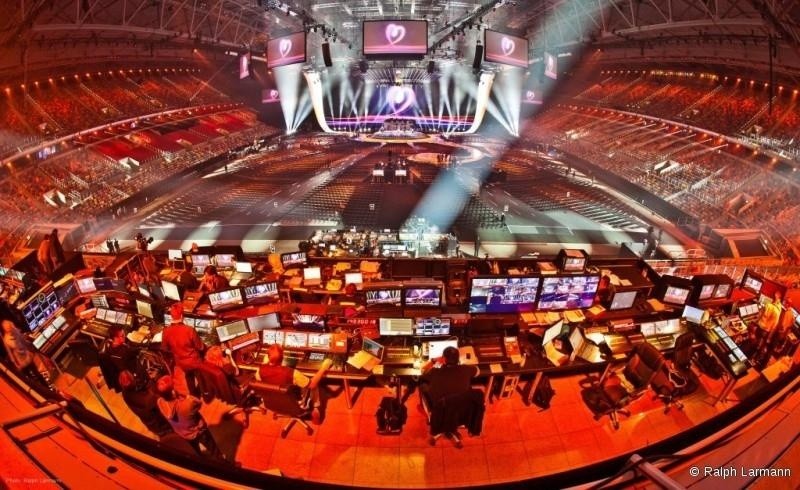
533;376;555;412
376;397;407;429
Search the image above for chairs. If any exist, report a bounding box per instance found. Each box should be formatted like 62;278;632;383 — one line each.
423;391;471;449
68;339;314;438
594;343;665;430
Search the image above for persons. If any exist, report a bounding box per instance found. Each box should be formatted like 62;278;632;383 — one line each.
365;149;458;185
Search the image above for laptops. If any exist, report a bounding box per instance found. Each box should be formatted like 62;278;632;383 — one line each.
345;273;363;291
428;337;458;368
362;336;384;372
568;326;600;363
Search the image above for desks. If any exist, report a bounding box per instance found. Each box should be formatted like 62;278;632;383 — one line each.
51;322;703;408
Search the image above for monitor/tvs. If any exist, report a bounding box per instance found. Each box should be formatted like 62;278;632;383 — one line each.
0;249;331;362
403;280;443;310
362;281;403;313
416;317;450;335
555;249;586;272
691;275;716;300
465;274;543;314
608;291;637;312
534;273;601;312
712;274;734;299
740;268;763;296
379;317;413;336
655;274;695;307
